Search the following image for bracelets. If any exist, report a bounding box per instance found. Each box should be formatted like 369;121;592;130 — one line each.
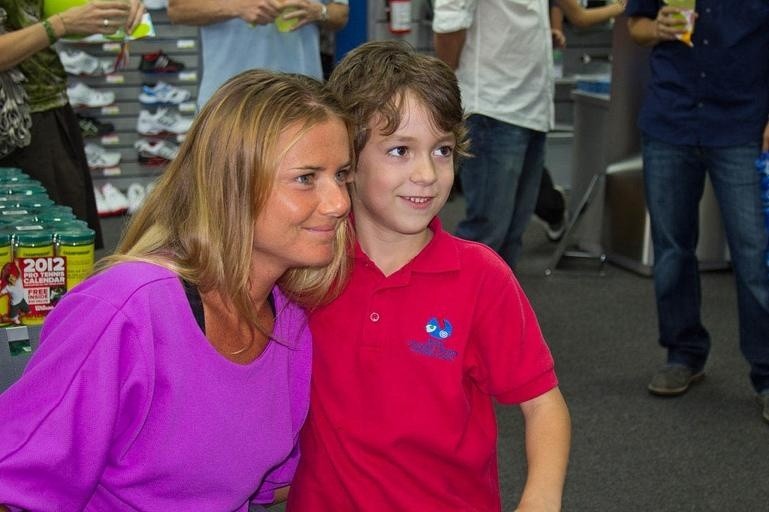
321;5;328;22
56;12;70;38
41;20;57;44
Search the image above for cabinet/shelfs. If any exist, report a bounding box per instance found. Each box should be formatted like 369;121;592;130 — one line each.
544;22;615;146
50;0;204;220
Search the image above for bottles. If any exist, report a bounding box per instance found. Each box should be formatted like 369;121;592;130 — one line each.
0;167;96;355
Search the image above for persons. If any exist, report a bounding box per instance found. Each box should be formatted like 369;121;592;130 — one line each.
625;0;769;423
435;1;554;269
166;0;350;116
1;68;352;511
284;40;570;512
0;260;32;324
532;1;626;242
1;0;147;262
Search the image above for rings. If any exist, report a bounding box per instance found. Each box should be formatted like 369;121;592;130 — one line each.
104;19;111;28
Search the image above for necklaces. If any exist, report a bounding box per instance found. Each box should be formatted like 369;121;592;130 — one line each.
200;303;256;354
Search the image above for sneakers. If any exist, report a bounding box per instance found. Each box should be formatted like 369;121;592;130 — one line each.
94;184;145;216
59;50;121;167
649;364;705;395
134;52;193;160
546;186;567;243
